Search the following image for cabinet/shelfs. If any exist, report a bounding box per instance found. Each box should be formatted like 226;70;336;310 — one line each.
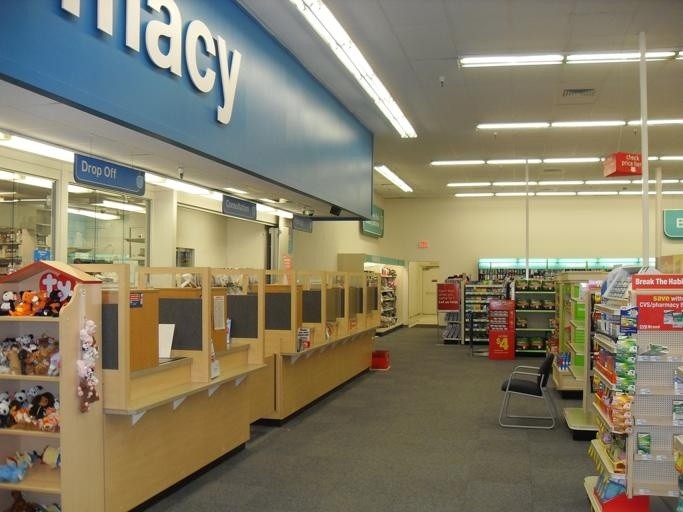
546;279;589;390
464;280;515;346
562;288;595;443
336;252;406;338
600;269;661;308
0;258;105;512
436;272;464;346
555;270;608;301
583;289;627;512
515;276;556;353
478;258;551;279
547;256;656;277
626;272;683;500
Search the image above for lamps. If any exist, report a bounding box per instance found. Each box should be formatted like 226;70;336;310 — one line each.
454;190;683;198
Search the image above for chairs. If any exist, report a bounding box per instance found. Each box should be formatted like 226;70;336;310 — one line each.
498;353;559;430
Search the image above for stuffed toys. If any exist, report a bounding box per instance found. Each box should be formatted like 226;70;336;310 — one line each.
76;320;100;413
0;386;60;433
1;335;61;376
0;289;70;318
